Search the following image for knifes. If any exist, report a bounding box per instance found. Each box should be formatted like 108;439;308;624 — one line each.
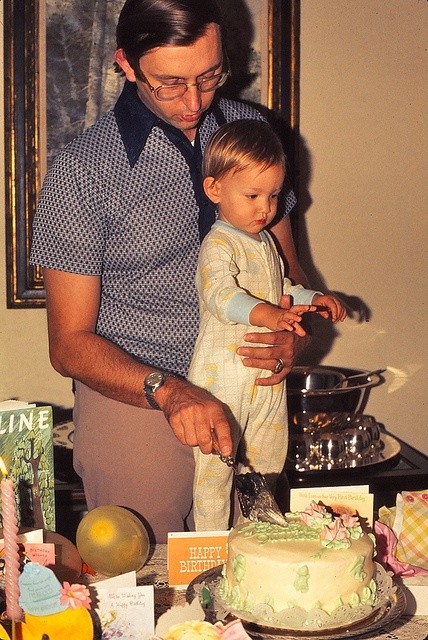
210;427;287;526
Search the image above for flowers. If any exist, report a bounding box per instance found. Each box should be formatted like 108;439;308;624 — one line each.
59;580;92;612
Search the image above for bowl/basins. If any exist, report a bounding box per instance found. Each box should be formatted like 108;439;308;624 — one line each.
286;368;349;389
286;365;381;421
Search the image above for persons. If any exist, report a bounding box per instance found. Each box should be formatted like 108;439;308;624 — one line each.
183;118;349;533
28;0;326;544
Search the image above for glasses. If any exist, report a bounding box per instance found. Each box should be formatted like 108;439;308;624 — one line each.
126;43;230;100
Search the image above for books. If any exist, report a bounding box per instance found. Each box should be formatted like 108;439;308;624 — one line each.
1;398;57;534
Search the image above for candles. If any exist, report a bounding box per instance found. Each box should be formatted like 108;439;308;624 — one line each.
0;456;23;640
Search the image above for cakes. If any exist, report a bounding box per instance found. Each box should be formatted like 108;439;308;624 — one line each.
221;503;381;627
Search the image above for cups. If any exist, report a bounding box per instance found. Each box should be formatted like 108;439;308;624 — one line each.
355;416;381;443
287;432;314;463
341;427;370;463
314;431;346;466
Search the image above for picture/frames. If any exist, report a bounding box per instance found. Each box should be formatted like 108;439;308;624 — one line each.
3;0;300;310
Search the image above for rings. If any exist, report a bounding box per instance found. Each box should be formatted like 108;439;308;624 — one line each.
274;359;285;375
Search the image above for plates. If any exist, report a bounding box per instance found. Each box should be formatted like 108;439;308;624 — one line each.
285;431;402;474
185;563;407;640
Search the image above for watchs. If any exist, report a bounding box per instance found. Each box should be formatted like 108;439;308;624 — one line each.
142;371;177;412
